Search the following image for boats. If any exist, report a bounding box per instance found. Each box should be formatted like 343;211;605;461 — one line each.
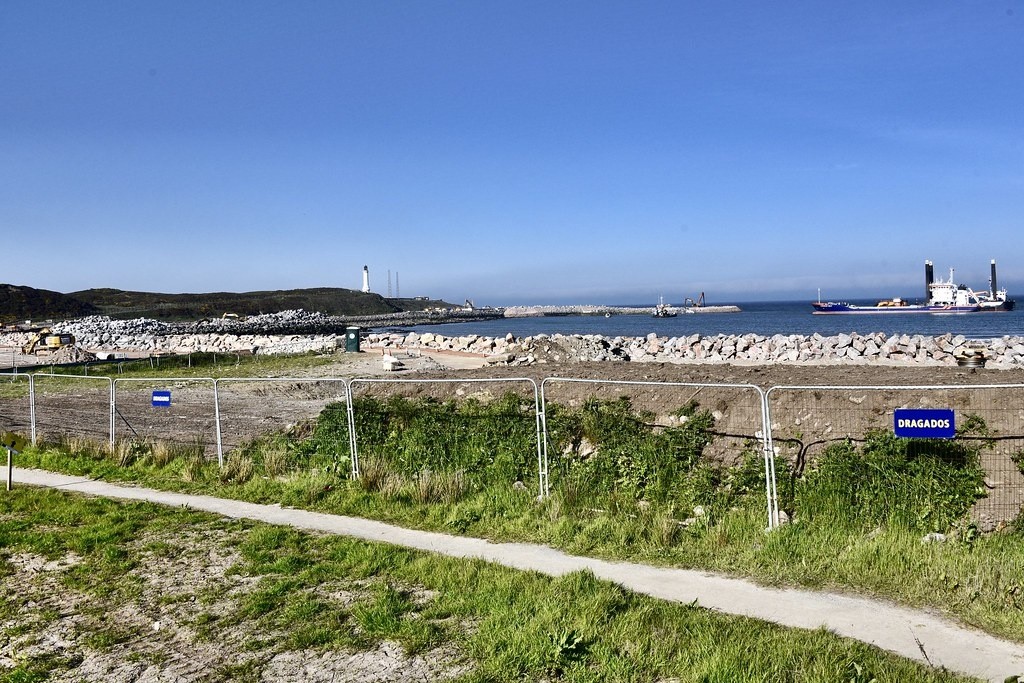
652;293;677;318
810;264;982;315
923;258;1016;312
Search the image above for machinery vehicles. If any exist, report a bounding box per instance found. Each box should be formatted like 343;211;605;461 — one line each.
19;327;75;356
683;292;706;309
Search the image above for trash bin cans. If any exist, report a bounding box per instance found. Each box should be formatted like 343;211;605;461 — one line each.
346;326;360;352
